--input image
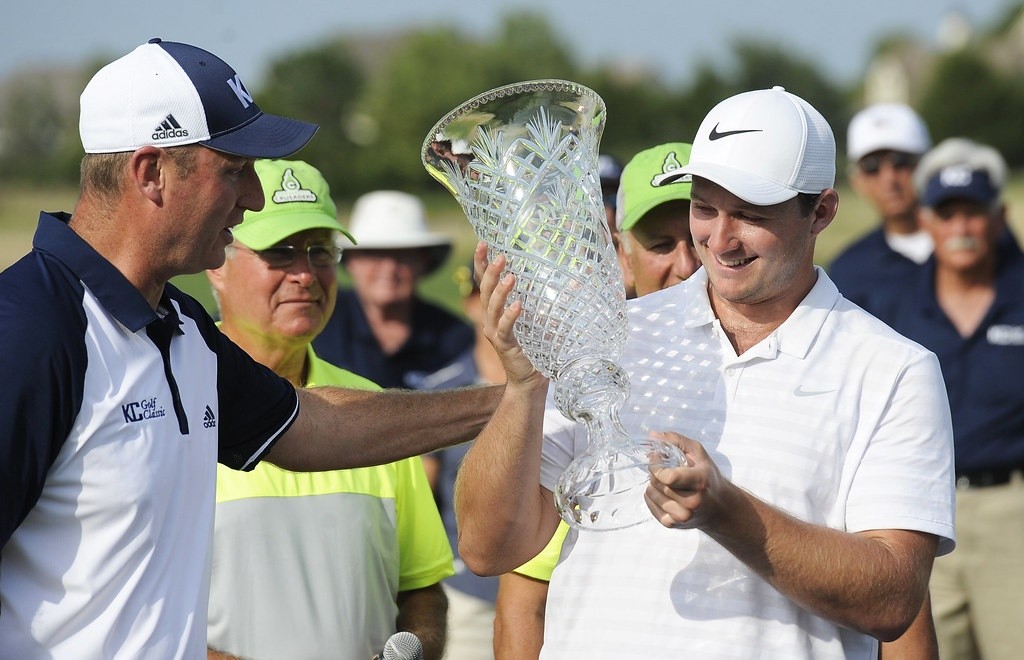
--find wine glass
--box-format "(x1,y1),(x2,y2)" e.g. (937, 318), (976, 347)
(421, 79), (688, 533)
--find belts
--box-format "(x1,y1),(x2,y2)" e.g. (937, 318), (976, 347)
(953, 468), (1021, 487)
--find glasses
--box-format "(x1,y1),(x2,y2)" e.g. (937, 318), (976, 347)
(225, 243), (344, 268)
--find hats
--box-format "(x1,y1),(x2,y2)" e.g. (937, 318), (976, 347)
(656, 86), (835, 205)
(846, 104), (929, 164)
(78, 37), (320, 160)
(615, 143), (692, 231)
(919, 166), (997, 206)
(336, 191), (456, 276)
(228, 159), (357, 252)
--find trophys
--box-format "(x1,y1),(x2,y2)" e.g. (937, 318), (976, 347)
(420, 78), (690, 534)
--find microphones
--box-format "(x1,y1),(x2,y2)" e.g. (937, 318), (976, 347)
(372, 631), (422, 660)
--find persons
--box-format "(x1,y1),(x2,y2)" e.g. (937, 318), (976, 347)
(452, 85), (956, 660)
(0, 37), (507, 660)
(207, 159), (455, 660)
(310, 141), (939, 660)
(856, 138), (1023, 660)
(824, 104), (1024, 311)
